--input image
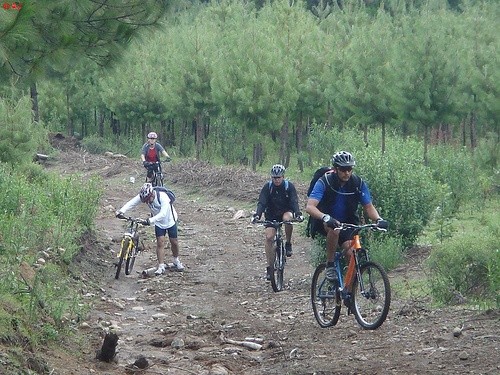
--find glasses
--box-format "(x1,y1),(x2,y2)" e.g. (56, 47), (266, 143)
(336, 166), (352, 172)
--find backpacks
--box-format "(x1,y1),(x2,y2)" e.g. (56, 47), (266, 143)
(153, 187), (176, 204)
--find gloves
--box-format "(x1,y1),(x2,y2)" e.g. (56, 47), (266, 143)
(294, 212), (304, 220)
(251, 213), (260, 223)
(115, 210), (123, 218)
(321, 214), (341, 230)
(375, 218), (387, 229)
(142, 218), (149, 226)
(143, 161), (149, 166)
(167, 156), (171, 161)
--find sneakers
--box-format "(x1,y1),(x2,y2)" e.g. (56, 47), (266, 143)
(325, 266), (338, 280)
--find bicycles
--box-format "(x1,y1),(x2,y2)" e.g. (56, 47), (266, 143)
(256, 219), (302, 292)
(114, 215), (150, 280)
(311, 223), (391, 330)
(146, 160), (170, 187)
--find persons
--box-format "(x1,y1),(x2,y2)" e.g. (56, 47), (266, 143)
(306, 151), (388, 295)
(118, 184), (185, 273)
(141, 132), (171, 181)
(250, 165), (304, 281)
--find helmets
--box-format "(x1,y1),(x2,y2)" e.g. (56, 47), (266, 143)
(271, 163), (285, 178)
(147, 132), (157, 139)
(140, 183), (152, 201)
(332, 151), (356, 167)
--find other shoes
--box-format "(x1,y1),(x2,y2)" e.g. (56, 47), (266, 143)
(285, 243), (293, 256)
(266, 268), (270, 279)
(155, 266), (165, 275)
(173, 260), (184, 270)
